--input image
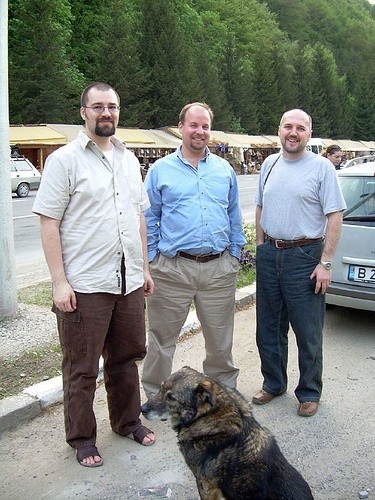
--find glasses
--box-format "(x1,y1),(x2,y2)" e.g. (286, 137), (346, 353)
(328, 145), (339, 153)
(84, 105), (121, 114)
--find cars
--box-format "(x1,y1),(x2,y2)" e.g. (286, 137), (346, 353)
(323, 155), (375, 317)
(10, 155), (41, 198)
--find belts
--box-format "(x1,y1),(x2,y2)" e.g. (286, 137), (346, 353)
(264, 233), (323, 249)
(179, 248), (228, 262)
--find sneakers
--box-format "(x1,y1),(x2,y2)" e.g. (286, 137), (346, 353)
(252, 388), (274, 405)
(298, 401), (319, 416)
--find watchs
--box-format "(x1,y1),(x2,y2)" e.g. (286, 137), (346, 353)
(319, 260), (333, 270)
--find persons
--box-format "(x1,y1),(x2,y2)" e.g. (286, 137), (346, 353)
(141, 102), (248, 401)
(32, 83), (155, 468)
(252, 109), (347, 417)
(258, 150), (264, 165)
(321, 144), (343, 165)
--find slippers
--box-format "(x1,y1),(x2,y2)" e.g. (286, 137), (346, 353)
(127, 425), (157, 446)
(75, 444), (103, 467)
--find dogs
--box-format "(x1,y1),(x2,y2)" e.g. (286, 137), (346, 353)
(137, 362), (315, 500)
